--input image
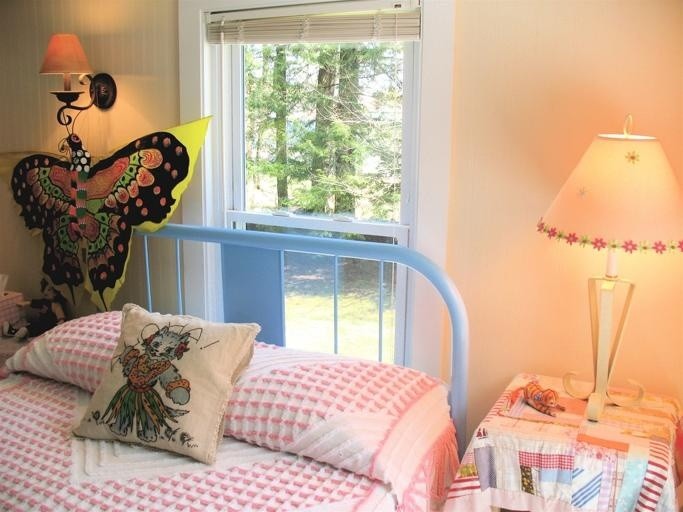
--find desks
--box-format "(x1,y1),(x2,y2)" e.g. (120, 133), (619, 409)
(473, 375), (681, 512)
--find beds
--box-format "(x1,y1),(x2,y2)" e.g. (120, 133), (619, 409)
(2, 221), (472, 512)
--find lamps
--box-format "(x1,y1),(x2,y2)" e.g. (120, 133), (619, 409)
(537, 116), (683, 409)
(39, 31), (117, 126)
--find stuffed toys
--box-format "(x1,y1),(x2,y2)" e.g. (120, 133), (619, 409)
(525, 382), (565, 416)
(3, 278), (66, 340)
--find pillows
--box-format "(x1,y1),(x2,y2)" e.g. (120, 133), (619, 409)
(67, 304), (264, 464)
